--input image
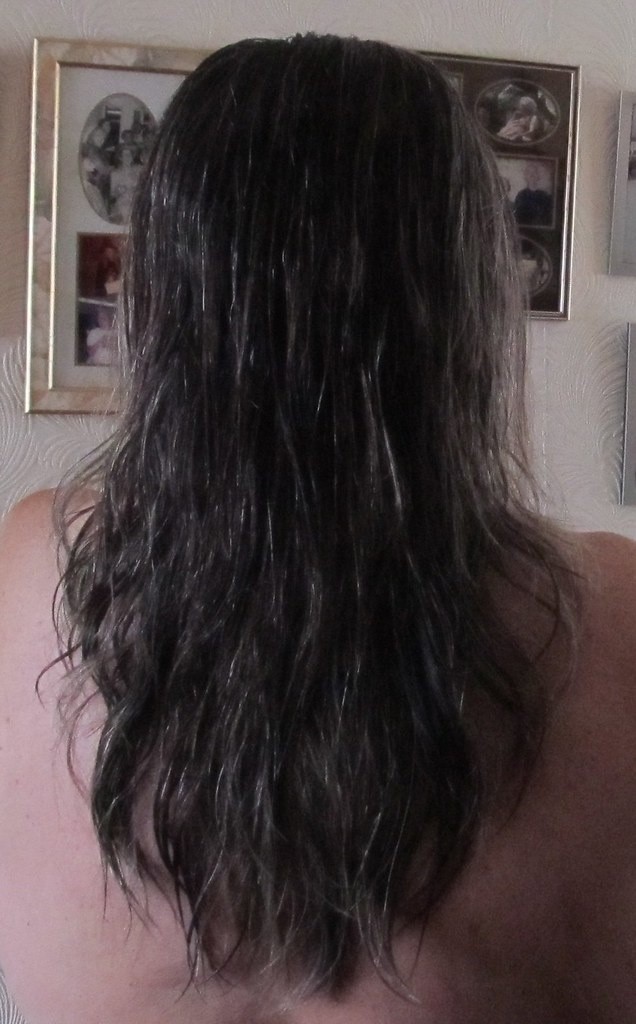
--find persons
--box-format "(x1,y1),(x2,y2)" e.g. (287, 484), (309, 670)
(83, 103), (159, 369)
(2, 31), (635, 1024)
(443, 71), (556, 307)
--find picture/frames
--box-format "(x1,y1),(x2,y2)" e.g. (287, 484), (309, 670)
(414, 45), (584, 321)
(24, 36), (215, 412)
